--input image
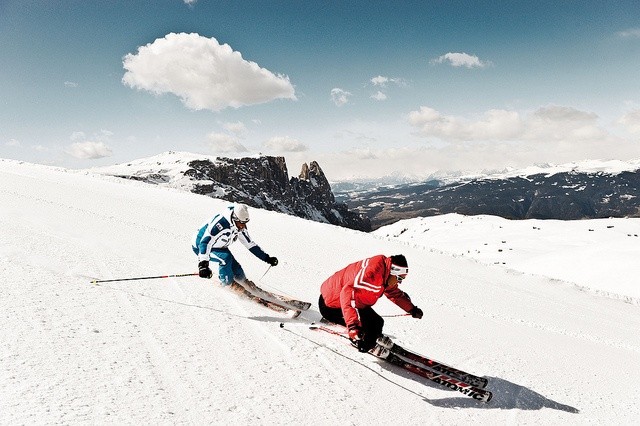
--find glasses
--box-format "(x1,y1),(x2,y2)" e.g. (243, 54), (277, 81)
(397, 275), (404, 281)
(232, 216), (246, 224)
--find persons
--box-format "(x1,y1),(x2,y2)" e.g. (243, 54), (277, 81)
(318, 254), (423, 358)
(191, 204), (278, 294)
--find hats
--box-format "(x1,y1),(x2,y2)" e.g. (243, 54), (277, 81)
(233, 204), (249, 221)
(390, 254), (408, 276)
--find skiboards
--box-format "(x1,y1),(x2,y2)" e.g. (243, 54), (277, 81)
(311, 313), (494, 405)
(210, 267), (312, 319)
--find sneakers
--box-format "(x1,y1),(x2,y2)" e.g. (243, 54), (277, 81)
(228, 281), (246, 293)
(368, 343), (390, 359)
(240, 278), (258, 290)
(376, 334), (394, 349)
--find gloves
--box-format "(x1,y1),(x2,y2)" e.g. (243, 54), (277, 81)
(266, 254), (278, 267)
(410, 306), (423, 319)
(347, 323), (365, 342)
(198, 260), (212, 279)
